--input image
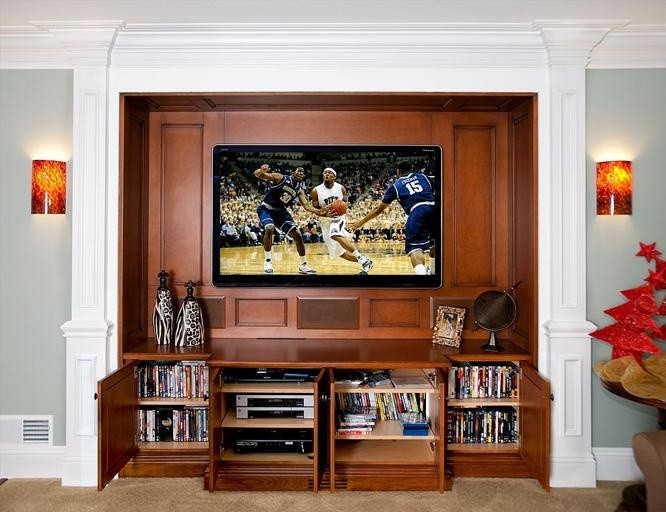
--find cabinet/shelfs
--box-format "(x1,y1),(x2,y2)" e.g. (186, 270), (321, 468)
(93, 90), (555, 495)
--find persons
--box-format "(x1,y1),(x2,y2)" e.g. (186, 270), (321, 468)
(215, 147), (438, 277)
(440, 316), (454, 338)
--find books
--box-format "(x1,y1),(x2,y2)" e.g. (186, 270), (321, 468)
(334, 361), (521, 445)
(134, 359), (209, 443)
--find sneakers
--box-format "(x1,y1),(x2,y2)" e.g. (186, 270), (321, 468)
(426, 267), (431, 275)
(358, 259), (373, 275)
(264, 258), (273, 273)
(298, 262), (317, 275)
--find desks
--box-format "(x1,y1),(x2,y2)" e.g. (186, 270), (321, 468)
(599, 377), (665, 511)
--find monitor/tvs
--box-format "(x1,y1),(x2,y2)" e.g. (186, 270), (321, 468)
(212, 144), (442, 290)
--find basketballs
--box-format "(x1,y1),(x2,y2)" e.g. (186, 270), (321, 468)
(330, 200), (347, 216)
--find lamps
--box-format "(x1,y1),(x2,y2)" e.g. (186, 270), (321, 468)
(31, 159), (68, 215)
(595, 160), (634, 216)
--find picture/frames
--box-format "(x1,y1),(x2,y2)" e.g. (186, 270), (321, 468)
(430, 304), (466, 348)
